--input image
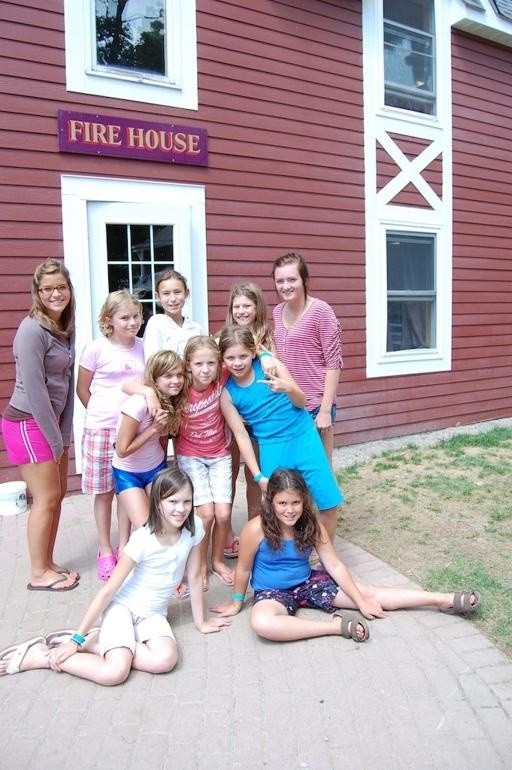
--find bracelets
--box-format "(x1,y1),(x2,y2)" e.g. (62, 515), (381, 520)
(232, 591), (245, 601)
(70, 633), (86, 648)
(259, 351), (272, 360)
(254, 473), (264, 482)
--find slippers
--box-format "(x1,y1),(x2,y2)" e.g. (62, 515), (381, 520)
(177, 536), (241, 600)
(25, 567), (80, 592)
(0, 627), (102, 676)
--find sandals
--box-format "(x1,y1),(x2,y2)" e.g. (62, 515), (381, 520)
(331, 609), (370, 644)
(440, 588), (483, 617)
(95, 545), (126, 581)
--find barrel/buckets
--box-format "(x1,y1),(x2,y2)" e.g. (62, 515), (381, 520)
(0, 480), (28, 515)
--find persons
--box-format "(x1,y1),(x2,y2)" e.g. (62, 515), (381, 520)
(271, 252), (346, 467)
(1, 257), (81, 592)
(74, 288), (146, 582)
(110, 349), (191, 601)
(219, 324), (345, 565)
(0, 467), (232, 687)
(119, 333), (280, 594)
(207, 467), (483, 643)
(221, 282), (276, 563)
(142, 269), (204, 458)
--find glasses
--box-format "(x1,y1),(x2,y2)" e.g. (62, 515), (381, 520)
(34, 284), (71, 296)
